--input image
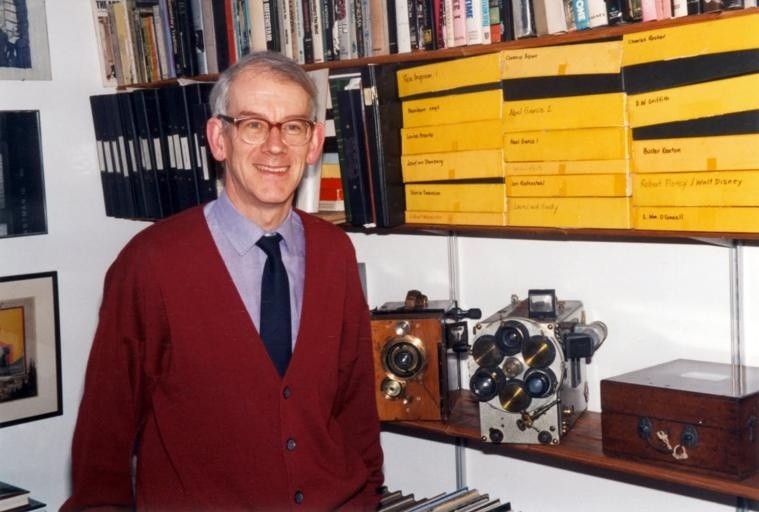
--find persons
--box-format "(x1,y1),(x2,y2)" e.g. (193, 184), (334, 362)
(52, 52), (389, 512)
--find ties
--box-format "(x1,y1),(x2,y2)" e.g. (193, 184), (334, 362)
(256, 234), (292, 376)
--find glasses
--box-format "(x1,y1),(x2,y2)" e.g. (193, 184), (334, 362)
(218, 114), (315, 148)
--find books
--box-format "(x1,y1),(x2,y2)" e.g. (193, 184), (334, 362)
(0, 479), (31, 511)
(385, 1), (759, 54)
(93, 0), (402, 233)
(379, 485), (522, 511)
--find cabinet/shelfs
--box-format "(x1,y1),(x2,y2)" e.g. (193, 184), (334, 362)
(113, 4), (759, 507)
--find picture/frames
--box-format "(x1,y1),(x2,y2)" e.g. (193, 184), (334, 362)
(0, 271), (64, 428)
(0, 0), (53, 80)
(0, 109), (48, 239)
(90, 0), (118, 87)
